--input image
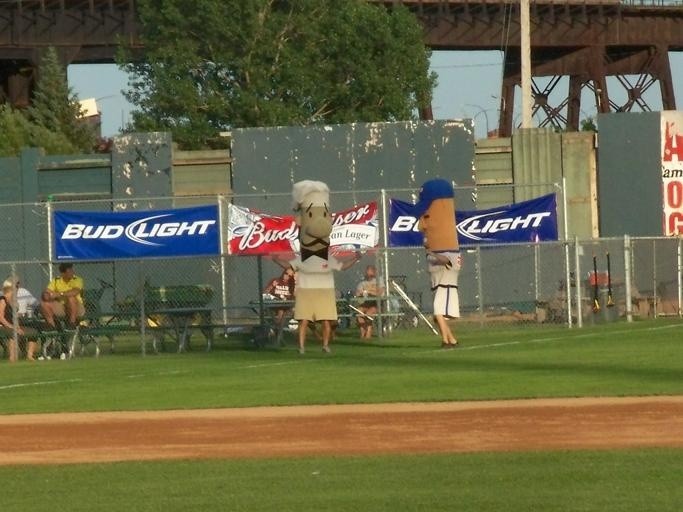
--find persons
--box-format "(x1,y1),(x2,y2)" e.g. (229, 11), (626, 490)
(39, 263), (86, 333)
(6, 275), (38, 319)
(353, 262), (384, 338)
(261, 264), (297, 346)
(411, 179), (461, 353)
(276, 178), (372, 353)
(0, 278), (38, 362)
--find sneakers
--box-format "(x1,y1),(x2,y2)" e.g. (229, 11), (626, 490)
(60, 352), (70, 359)
(441, 341), (449, 347)
(283, 271), (293, 279)
(451, 341), (460, 347)
(38, 354), (52, 361)
(297, 347), (304, 354)
(64, 326), (77, 332)
(41, 327), (58, 333)
(322, 345), (331, 353)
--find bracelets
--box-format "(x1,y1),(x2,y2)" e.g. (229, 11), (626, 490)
(59, 292), (64, 297)
